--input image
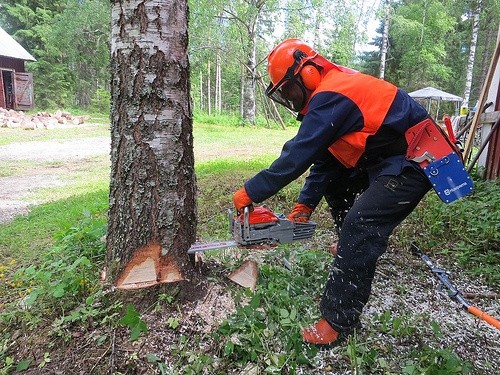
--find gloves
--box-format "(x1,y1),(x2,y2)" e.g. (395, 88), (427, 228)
(232, 188), (254, 215)
(287, 203), (313, 222)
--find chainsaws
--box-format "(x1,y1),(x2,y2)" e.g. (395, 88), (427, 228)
(187, 206), (317, 252)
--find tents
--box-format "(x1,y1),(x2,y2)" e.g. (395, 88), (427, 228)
(407, 86), (464, 123)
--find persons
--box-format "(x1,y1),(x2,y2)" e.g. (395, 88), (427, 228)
(232, 38), (476, 344)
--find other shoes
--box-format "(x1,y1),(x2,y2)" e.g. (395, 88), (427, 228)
(302, 316), (363, 350)
(330, 243), (338, 255)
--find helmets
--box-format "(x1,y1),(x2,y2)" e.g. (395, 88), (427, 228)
(268, 38), (339, 92)
